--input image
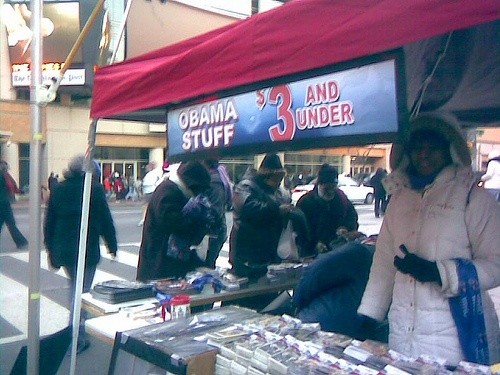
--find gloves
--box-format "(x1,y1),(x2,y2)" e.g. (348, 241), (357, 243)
(394, 243), (439, 282)
(355, 314), (378, 341)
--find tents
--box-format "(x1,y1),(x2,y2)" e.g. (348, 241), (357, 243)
(69, 0), (500, 374)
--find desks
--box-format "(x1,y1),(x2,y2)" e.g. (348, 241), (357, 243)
(82, 264), (296, 316)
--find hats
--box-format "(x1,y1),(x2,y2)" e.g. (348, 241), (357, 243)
(67, 153), (100, 178)
(259, 152), (286, 176)
(318, 163), (338, 184)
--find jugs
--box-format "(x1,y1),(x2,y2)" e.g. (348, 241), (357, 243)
(161, 294), (191, 321)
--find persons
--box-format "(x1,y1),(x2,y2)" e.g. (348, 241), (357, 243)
(381, 169), (392, 215)
(478, 150), (500, 202)
(228, 153), (305, 269)
(136, 161), (163, 227)
(136, 159), (225, 283)
(291, 233), (389, 344)
(202, 157), (233, 270)
(357, 110), (500, 371)
(161, 162), (171, 187)
(48, 172), (59, 195)
(43, 154), (119, 357)
(295, 163), (359, 253)
(0, 160), (29, 249)
(101, 172), (143, 202)
(370, 168), (387, 218)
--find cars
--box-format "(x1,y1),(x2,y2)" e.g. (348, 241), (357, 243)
(290, 176), (375, 207)
